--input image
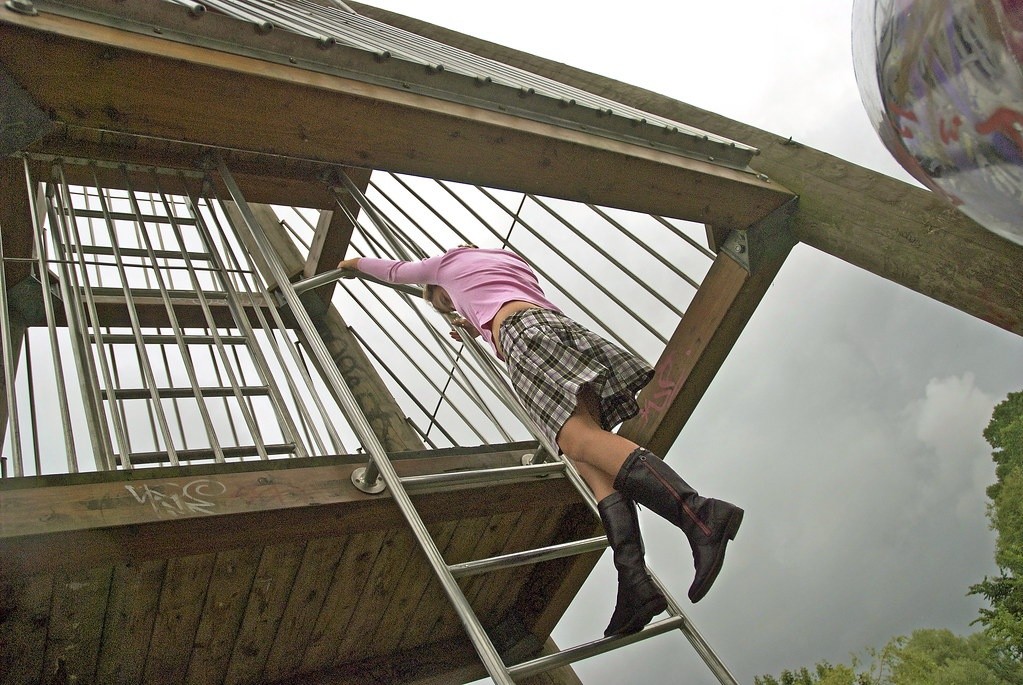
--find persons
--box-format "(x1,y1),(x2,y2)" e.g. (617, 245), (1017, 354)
(337, 244), (744, 637)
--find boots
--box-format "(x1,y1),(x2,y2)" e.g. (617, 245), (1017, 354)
(597, 492), (669, 637)
(612, 444), (744, 605)
